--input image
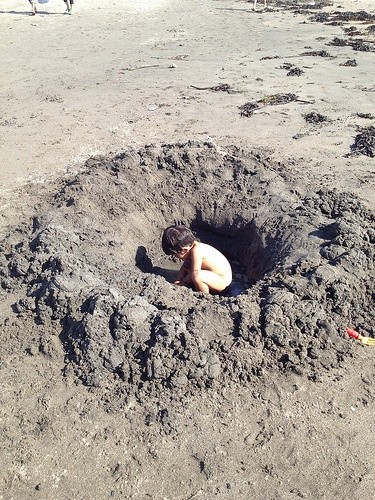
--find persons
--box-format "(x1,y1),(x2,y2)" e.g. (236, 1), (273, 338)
(161, 227), (233, 295)
(29, 0), (37, 15)
(63, 0), (74, 15)
